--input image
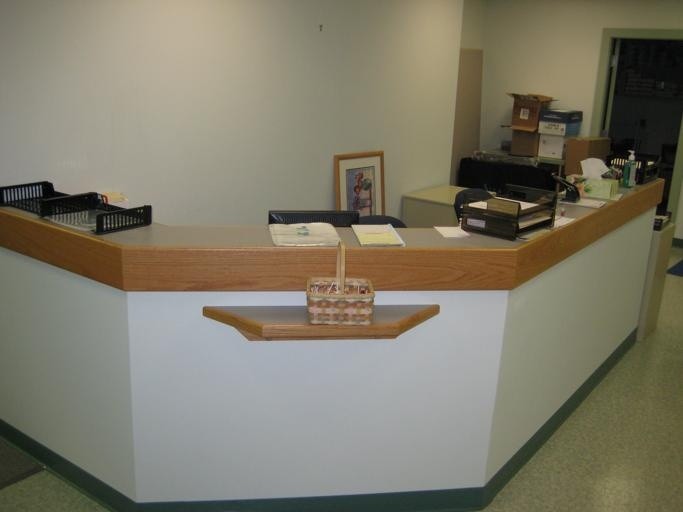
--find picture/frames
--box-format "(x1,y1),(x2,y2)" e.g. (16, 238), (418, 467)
(334, 151), (385, 219)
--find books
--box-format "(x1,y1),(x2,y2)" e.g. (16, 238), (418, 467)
(266, 220), (340, 247)
(349, 221), (406, 247)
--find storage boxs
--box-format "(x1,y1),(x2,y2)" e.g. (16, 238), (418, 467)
(565, 136), (611, 176)
(512, 128), (540, 157)
(537, 108), (583, 136)
(538, 133), (567, 159)
(507, 93), (558, 133)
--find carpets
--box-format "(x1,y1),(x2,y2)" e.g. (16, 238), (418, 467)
(0, 438), (42, 490)
(667, 258), (683, 278)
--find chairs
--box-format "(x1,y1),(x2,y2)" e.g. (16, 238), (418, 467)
(359, 215), (406, 228)
(453, 188), (493, 224)
(506, 165), (555, 201)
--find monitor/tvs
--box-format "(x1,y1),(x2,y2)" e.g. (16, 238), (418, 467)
(269, 211), (359, 227)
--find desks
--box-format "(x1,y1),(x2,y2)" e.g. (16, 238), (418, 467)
(636, 223), (675, 341)
(404, 183), (496, 227)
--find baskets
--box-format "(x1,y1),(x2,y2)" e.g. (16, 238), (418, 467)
(305, 240), (376, 327)
